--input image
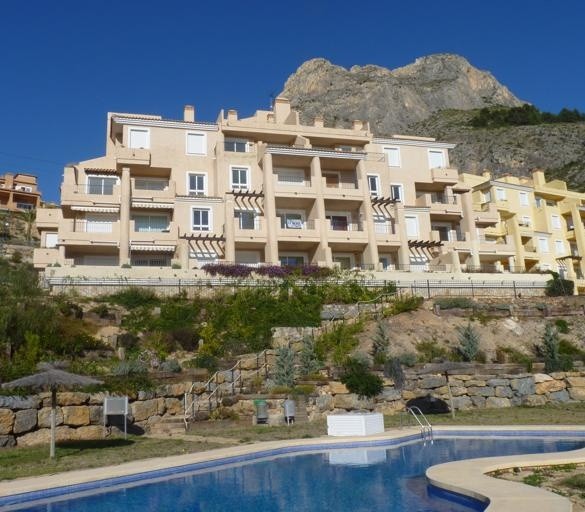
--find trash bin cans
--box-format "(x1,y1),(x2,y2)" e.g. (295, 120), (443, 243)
(283, 400), (296, 423)
(252, 399), (269, 424)
